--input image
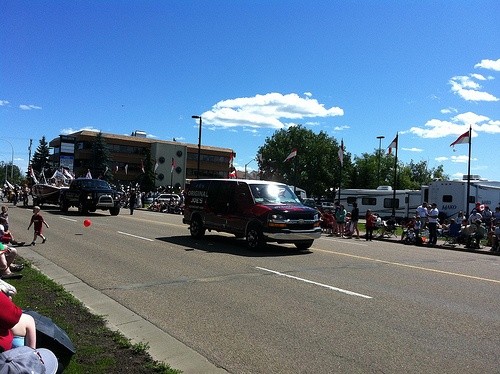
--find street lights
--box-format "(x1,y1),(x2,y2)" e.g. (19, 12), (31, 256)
(376, 136), (385, 186)
(245, 159), (256, 179)
(191, 116), (202, 179)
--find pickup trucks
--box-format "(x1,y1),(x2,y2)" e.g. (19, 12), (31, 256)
(58, 178), (121, 216)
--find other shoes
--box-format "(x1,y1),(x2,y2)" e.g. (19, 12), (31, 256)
(30, 242), (37, 246)
(10, 263), (24, 272)
(1, 272), (23, 280)
(12, 241), (25, 246)
(42, 237), (48, 244)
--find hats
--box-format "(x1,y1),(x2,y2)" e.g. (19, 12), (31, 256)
(0, 345), (59, 374)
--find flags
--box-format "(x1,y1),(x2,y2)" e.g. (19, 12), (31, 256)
(6, 180), (14, 189)
(338, 143), (343, 167)
(104, 157), (175, 175)
(283, 146), (297, 162)
(228, 152), (233, 168)
(385, 137), (397, 154)
(229, 171), (236, 177)
(30, 167), (92, 183)
(449, 131), (470, 146)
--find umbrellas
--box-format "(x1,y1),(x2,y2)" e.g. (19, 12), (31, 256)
(22, 311), (76, 354)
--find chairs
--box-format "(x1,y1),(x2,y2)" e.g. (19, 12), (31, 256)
(438, 229), (456, 244)
(372, 223), (395, 239)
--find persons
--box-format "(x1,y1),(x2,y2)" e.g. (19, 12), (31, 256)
(129, 190), (135, 215)
(365, 210), (397, 242)
(109, 182), (145, 209)
(229, 164), (238, 179)
(35, 178), (71, 187)
(27, 206), (49, 246)
(314, 202), (359, 240)
(0, 205), (25, 280)
(147, 186), (184, 215)
(0, 184), (30, 207)
(401, 200), (500, 253)
(0, 278), (58, 374)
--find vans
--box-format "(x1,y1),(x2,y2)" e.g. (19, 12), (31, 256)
(182, 178), (323, 253)
(315, 201), (336, 210)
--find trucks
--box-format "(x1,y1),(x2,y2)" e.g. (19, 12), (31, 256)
(334, 185), (420, 219)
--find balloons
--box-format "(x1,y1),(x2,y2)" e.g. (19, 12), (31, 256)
(84, 220), (91, 227)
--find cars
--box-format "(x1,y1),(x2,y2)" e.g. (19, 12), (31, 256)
(145, 194), (181, 205)
